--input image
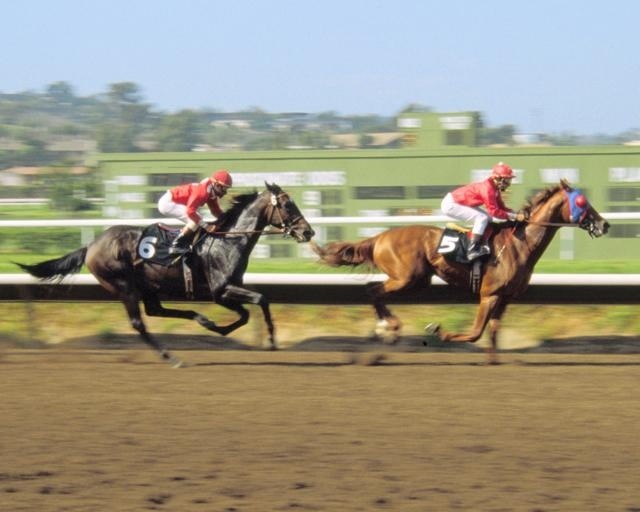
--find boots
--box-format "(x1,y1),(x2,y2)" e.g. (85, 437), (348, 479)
(168, 226), (193, 254)
(466, 234), (488, 261)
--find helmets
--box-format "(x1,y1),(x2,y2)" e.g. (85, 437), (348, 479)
(491, 162), (516, 178)
(209, 171), (232, 187)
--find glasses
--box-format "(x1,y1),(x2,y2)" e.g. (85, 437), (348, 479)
(216, 183), (227, 193)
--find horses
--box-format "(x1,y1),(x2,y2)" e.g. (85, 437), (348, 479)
(307, 176), (612, 355)
(12, 179), (315, 358)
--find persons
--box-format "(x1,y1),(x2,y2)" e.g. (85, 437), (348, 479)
(155, 168), (233, 253)
(440, 160), (525, 262)
